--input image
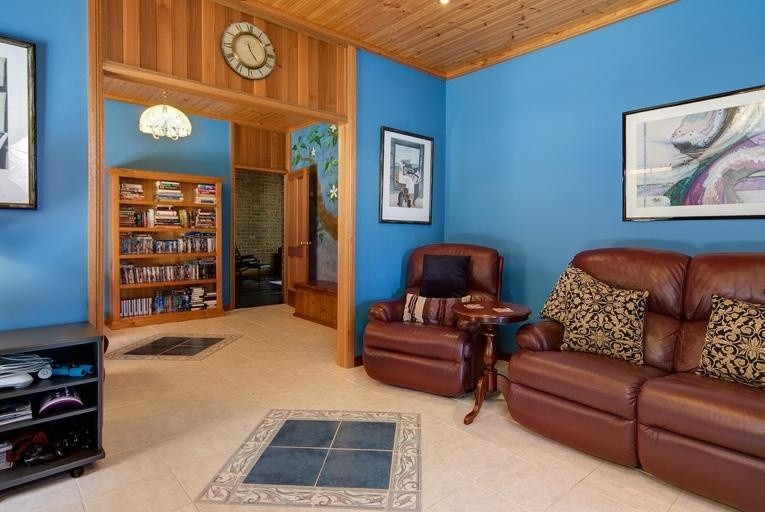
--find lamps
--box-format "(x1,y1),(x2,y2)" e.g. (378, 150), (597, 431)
(139, 91), (192, 140)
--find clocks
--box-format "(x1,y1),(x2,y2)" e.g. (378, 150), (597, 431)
(220, 21), (275, 80)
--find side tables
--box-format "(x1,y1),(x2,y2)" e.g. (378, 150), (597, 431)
(451, 301), (531, 425)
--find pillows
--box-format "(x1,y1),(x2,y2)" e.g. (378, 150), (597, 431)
(695, 294), (765, 390)
(560, 279), (650, 366)
(419, 254), (472, 298)
(401, 292), (471, 327)
(536, 264), (614, 324)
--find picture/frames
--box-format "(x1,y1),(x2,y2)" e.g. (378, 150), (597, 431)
(378, 126), (434, 225)
(0, 35), (38, 211)
(622, 84), (765, 221)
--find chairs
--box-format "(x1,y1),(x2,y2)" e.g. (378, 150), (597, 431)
(362, 244), (504, 399)
(235, 242), (261, 292)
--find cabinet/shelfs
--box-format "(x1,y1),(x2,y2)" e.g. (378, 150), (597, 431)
(104, 167), (228, 330)
(294, 281), (337, 330)
(0, 320), (104, 495)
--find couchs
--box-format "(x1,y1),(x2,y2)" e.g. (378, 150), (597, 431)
(506, 248), (765, 512)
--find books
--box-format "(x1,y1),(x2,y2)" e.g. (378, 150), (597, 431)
(119, 179), (217, 318)
(0, 441), (15, 470)
(0, 400), (33, 426)
(241, 254), (271, 269)
